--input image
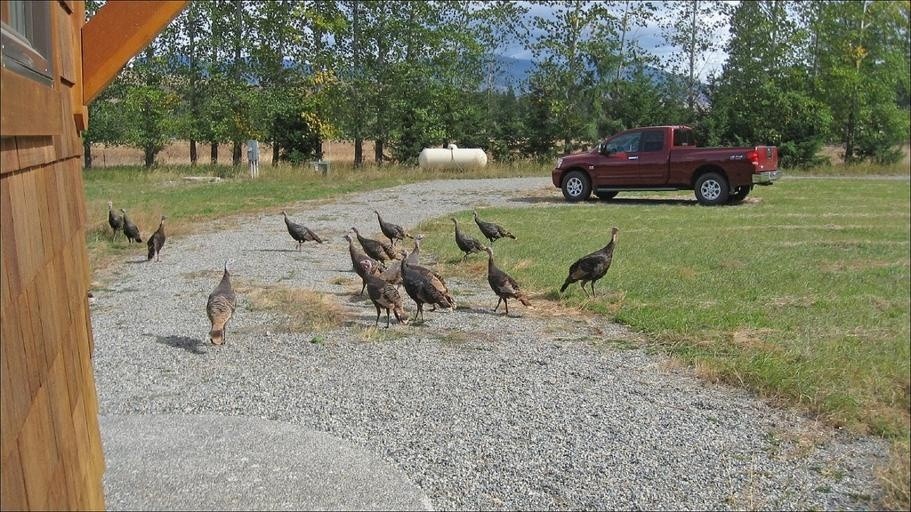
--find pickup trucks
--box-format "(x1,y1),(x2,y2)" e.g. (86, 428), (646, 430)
(551, 124), (778, 205)
